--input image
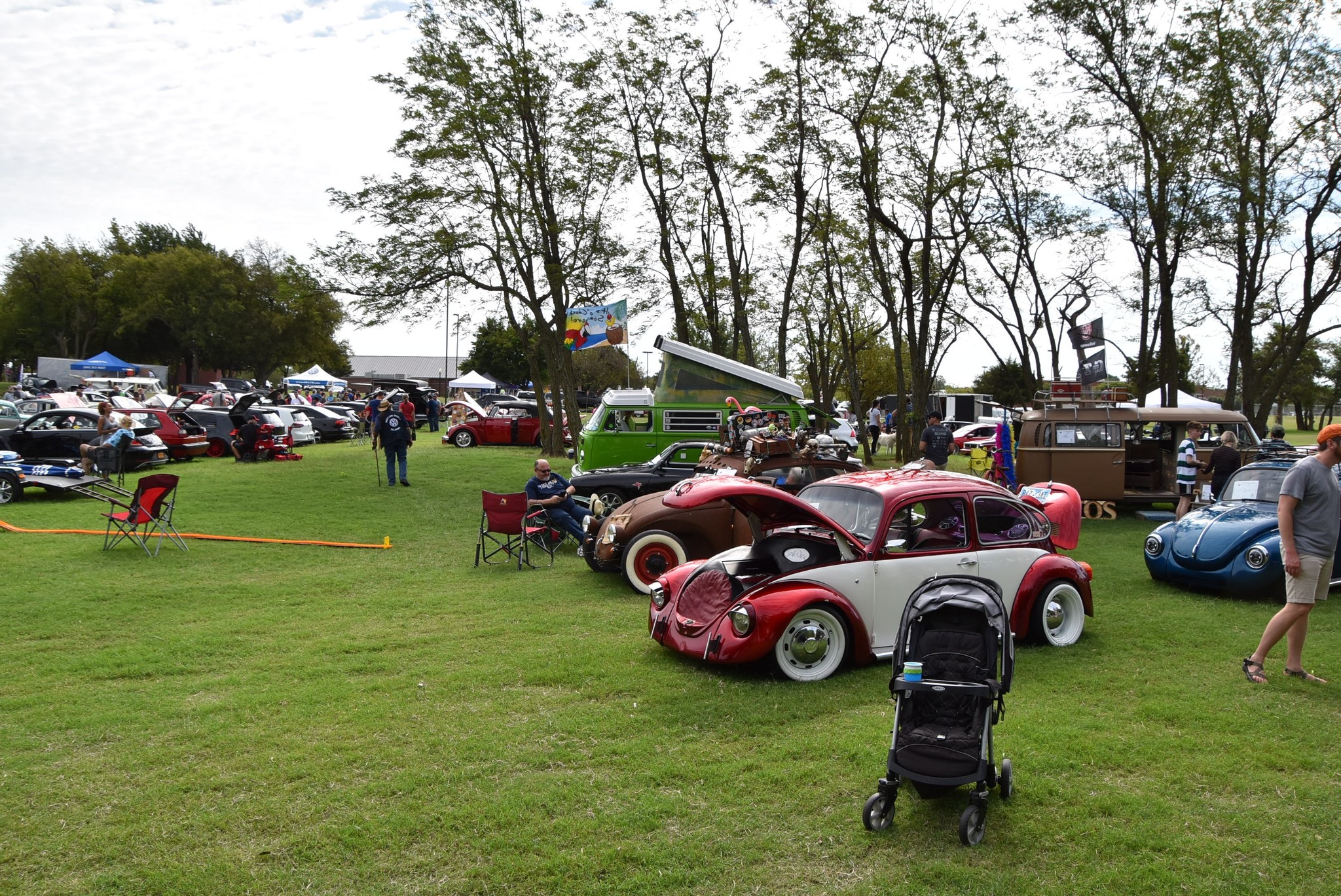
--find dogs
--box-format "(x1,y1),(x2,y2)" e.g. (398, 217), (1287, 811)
(870, 431), (902, 456)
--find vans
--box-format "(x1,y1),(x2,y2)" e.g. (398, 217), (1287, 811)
(570, 334), (814, 479)
(1015, 390), (1263, 517)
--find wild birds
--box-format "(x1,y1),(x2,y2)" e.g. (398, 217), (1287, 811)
(725, 397), (762, 414)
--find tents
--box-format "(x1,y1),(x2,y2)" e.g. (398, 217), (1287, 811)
(449, 370), (496, 398)
(1110, 383), (1222, 409)
(70, 350), (140, 381)
(283, 364), (348, 395)
(480, 372), (519, 397)
(515, 379), (534, 387)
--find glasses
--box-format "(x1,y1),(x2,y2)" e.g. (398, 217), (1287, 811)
(928, 416), (934, 420)
(305, 393), (308, 394)
(324, 395), (326, 396)
(536, 468), (552, 473)
(885, 411), (887, 413)
(345, 395), (349, 396)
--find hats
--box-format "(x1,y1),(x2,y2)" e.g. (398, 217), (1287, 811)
(15, 385), (22, 389)
(1271, 424), (1285, 434)
(906, 395), (911, 400)
(118, 387), (122, 391)
(340, 388), (353, 394)
(885, 409), (890, 412)
(377, 399), (393, 412)
(217, 386), (223, 391)
(42, 387), (47, 391)
(82, 380), (87, 382)
(106, 380), (112, 382)
(925, 411), (943, 420)
(590, 493), (605, 519)
(247, 415), (260, 421)
(118, 415), (136, 431)
(1316, 424), (1341, 445)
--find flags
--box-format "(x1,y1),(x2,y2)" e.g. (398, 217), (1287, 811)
(1066, 317), (1105, 350)
(1076, 349), (1107, 385)
(563, 299), (628, 351)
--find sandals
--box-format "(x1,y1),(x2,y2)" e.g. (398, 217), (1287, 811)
(1242, 655), (1269, 685)
(1282, 667), (1328, 685)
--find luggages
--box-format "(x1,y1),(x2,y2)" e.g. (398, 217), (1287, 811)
(719, 409), (797, 458)
(1051, 381), (1128, 403)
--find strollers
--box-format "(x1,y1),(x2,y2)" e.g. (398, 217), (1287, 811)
(862, 572), (1016, 847)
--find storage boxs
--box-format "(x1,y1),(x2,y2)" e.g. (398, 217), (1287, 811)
(748, 433), (796, 457)
(1110, 387), (1128, 401)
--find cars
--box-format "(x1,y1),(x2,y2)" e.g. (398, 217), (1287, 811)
(647, 456), (1097, 683)
(0, 368), (591, 506)
(940, 421), (976, 434)
(1143, 442), (1341, 606)
(583, 447), (872, 596)
(947, 423), (1001, 456)
(960, 433), (1002, 456)
(796, 390), (1114, 456)
(441, 400), (569, 449)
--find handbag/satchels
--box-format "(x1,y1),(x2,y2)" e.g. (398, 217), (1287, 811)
(412, 427), (416, 441)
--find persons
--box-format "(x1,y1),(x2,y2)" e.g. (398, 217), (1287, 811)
(865, 421), (871, 437)
(426, 394), (438, 432)
(247, 385), (257, 394)
(230, 415), (263, 462)
(96, 402), (121, 483)
(210, 386), (228, 407)
(1243, 423), (1341, 684)
(1253, 424), (1298, 463)
(372, 399), (413, 487)
(271, 392), (286, 406)
(784, 466), (806, 483)
(364, 396), (374, 437)
(918, 411), (955, 471)
(433, 395), (442, 431)
(1175, 421), (1209, 522)
(891, 395), (914, 453)
(399, 394), (416, 449)
(297, 387), (371, 409)
(269, 387), (274, 392)
(884, 409), (893, 434)
(592, 404), (600, 414)
(80, 415), (136, 476)
(524, 458), (594, 557)
(2, 381), (146, 408)
(865, 399), (882, 455)
(623, 411), (637, 431)
(290, 391), (305, 405)
(1201, 430), (1242, 505)
(365, 391), (386, 450)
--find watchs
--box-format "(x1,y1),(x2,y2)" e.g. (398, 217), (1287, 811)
(566, 492), (570, 498)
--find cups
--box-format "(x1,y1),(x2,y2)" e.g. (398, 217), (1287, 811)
(903, 662), (923, 681)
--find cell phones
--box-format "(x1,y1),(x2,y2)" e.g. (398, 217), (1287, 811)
(559, 491), (567, 498)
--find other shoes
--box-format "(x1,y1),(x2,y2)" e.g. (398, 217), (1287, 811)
(400, 478), (410, 486)
(372, 445), (376, 450)
(389, 481), (395, 486)
(871, 453), (878, 456)
(104, 477), (113, 483)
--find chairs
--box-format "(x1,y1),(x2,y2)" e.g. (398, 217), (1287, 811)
(474, 490), (554, 571)
(909, 538), (962, 552)
(348, 422), (371, 445)
(91, 434), (132, 491)
(242, 439), (273, 462)
(968, 446), (995, 480)
(273, 426), (293, 454)
(100, 473), (189, 559)
(526, 502), (583, 554)
(863, 526), (910, 547)
